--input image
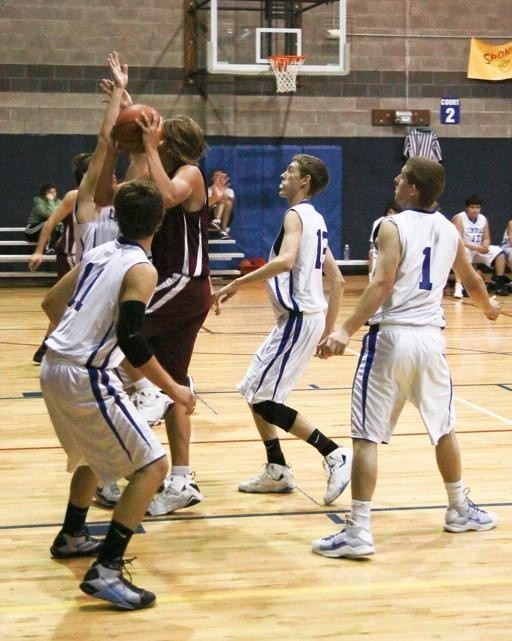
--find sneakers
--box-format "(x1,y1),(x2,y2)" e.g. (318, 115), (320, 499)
(79, 564), (157, 609)
(93, 479), (122, 508)
(49, 530), (105, 558)
(444, 506), (498, 532)
(45, 248), (55, 254)
(131, 388), (175, 428)
(494, 284), (508, 296)
(454, 284), (464, 298)
(146, 475), (202, 516)
(311, 525), (374, 558)
(207, 220), (230, 239)
(322, 447), (354, 504)
(238, 463), (296, 494)
(33, 346), (45, 361)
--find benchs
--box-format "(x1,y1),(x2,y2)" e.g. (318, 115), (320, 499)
(335, 259), (368, 266)
(0, 228), (245, 277)
(448, 272), (512, 283)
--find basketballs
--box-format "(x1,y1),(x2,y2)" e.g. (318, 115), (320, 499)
(116, 104), (161, 154)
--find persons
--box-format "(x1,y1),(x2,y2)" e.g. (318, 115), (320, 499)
(25, 50), (236, 609)
(209, 153), (354, 505)
(310, 158), (503, 560)
(450, 194), (508, 298)
(367, 203), (401, 282)
